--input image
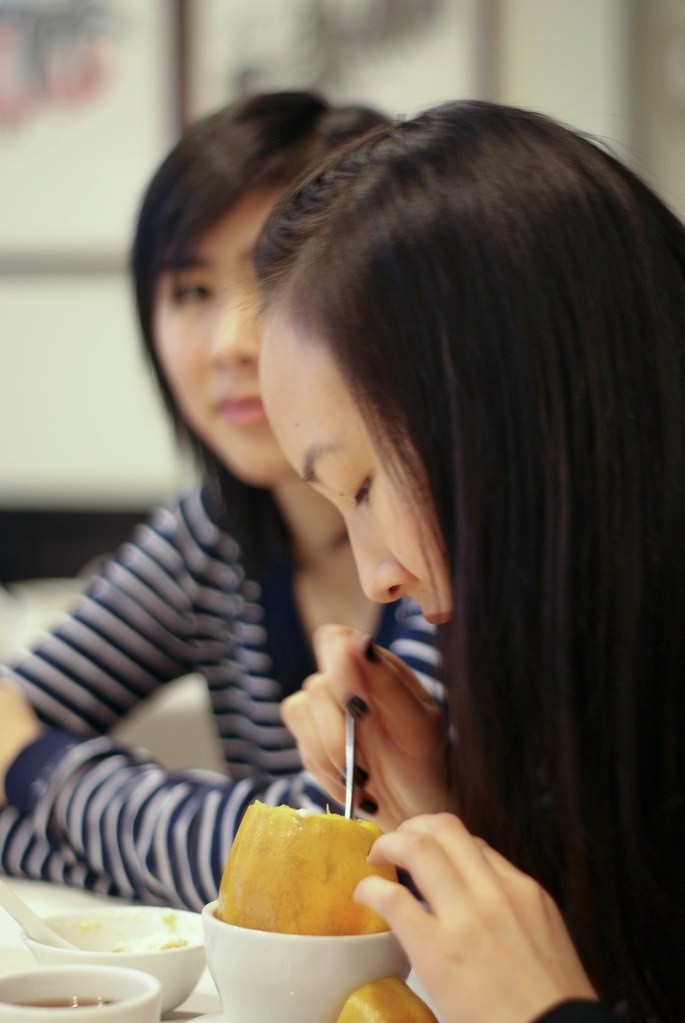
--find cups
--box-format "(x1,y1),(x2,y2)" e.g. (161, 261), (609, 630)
(0, 965), (161, 1023)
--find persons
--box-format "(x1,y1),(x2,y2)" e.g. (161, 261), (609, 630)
(0, 84), (441, 918)
(252, 95), (685, 1022)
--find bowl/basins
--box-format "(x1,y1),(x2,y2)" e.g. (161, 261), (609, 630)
(22, 907), (205, 1016)
(202, 900), (429, 1023)
